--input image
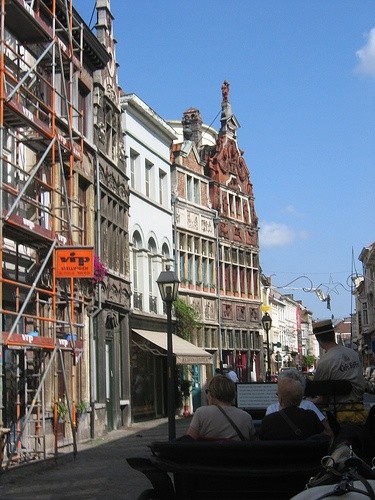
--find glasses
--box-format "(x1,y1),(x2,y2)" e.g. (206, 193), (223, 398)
(206, 389), (210, 394)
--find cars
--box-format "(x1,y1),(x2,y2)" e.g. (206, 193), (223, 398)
(362, 366), (375, 395)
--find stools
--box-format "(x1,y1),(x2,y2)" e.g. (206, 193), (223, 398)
(304, 379), (352, 419)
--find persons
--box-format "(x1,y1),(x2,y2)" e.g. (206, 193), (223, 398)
(266, 368), (332, 437)
(186, 375), (256, 441)
(308, 319), (364, 397)
(258, 377), (324, 440)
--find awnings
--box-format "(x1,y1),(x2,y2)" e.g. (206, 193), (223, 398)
(131, 328), (213, 364)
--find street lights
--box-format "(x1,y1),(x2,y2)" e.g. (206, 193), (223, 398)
(261, 311), (273, 383)
(156, 265), (182, 441)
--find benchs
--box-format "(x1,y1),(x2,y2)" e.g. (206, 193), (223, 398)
(149, 434), (333, 468)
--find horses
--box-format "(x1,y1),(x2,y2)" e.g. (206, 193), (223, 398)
(290, 404), (375, 500)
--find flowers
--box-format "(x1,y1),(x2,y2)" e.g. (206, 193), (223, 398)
(86, 254), (108, 289)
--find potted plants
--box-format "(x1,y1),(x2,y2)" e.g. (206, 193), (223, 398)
(49, 397), (87, 436)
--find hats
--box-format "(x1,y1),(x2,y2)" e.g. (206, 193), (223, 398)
(308, 319), (339, 335)
